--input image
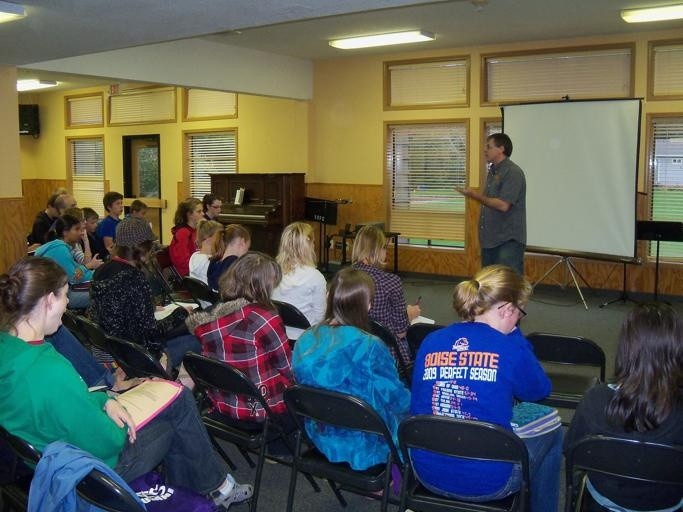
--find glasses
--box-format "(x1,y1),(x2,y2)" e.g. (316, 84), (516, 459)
(497, 301), (528, 321)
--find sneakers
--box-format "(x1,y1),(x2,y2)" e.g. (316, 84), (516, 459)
(210, 473), (253, 509)
(266, 454), (294, 465)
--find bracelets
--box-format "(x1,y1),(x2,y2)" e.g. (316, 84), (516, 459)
(482, 195), (487, 206)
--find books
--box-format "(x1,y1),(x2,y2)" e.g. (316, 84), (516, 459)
(511, 400), (562, 439)
(412, 314), (437, 328)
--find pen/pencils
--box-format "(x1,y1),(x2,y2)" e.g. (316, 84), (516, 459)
(415, 296), (422, 305)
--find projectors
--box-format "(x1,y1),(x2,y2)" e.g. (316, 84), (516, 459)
(352, 221), (386, 236)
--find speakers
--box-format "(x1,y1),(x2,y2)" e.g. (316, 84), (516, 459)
(18, 103), (40, 135)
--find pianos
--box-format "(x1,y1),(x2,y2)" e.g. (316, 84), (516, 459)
(215, 201), (281, 259)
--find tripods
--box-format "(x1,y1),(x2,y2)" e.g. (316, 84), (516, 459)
(598, 264), (640, 308)
(530, 256), (598, 311)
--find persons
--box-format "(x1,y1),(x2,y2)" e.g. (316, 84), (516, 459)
(289, 266), (411, 499)
(564, 301), (682, 511)
(25, 185), (335, 465)
(454, 132), (527, 332)
(410, 263), (564, 511)
(0, 256), (256, 507)
(348, 222), (448, 388)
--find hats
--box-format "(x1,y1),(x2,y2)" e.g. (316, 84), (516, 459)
(114, 214), (158, 248)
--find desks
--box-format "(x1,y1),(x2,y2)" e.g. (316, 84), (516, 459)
(325, 231), (401, 274)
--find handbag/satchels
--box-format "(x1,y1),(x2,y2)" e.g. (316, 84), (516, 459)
(513, 407), (562, 439)
(128, 469), (220, 512)
